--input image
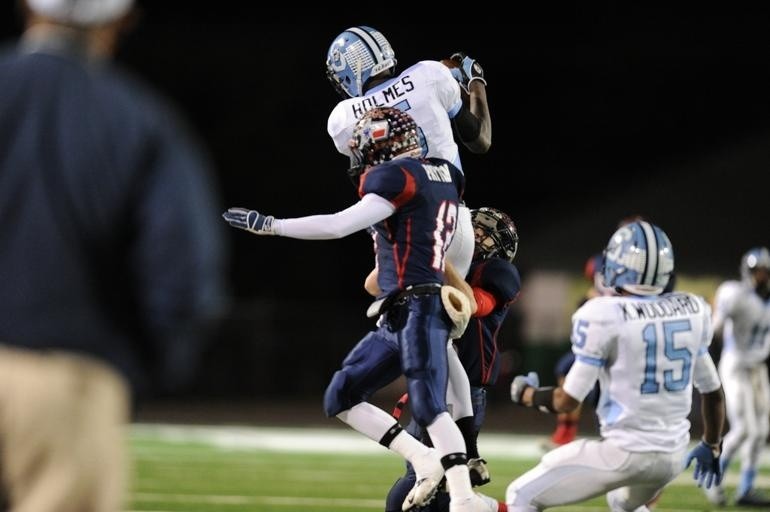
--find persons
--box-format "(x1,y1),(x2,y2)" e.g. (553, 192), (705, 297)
(324, 24), (493, 503)
(706, 246), (770, 505)
(540, 214), (663, 509)
(221, 105), (509, 511)
(0, 0), (226, 511)
(507, 221), (726, 512)
(362, 203), (522, 512)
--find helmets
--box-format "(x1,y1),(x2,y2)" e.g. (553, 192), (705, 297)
(604, 221), (675, 294)
(470, 206), (519, 263)
(348, 106), (423, 176)
(741, 248), (770, 290)
(324, 26), (399, 99)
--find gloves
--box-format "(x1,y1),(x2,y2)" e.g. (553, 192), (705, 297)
(511, 372), (539, 407)
(223, 207), (274, 236)
(684, 437), (723, 488)
(447, 52), (487, 95)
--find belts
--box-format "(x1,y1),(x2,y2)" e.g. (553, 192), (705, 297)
(389, 283), (441, 296)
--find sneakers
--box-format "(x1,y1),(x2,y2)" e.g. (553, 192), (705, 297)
(704, 476), (770, 508)
(401, 448), (507, 512)
